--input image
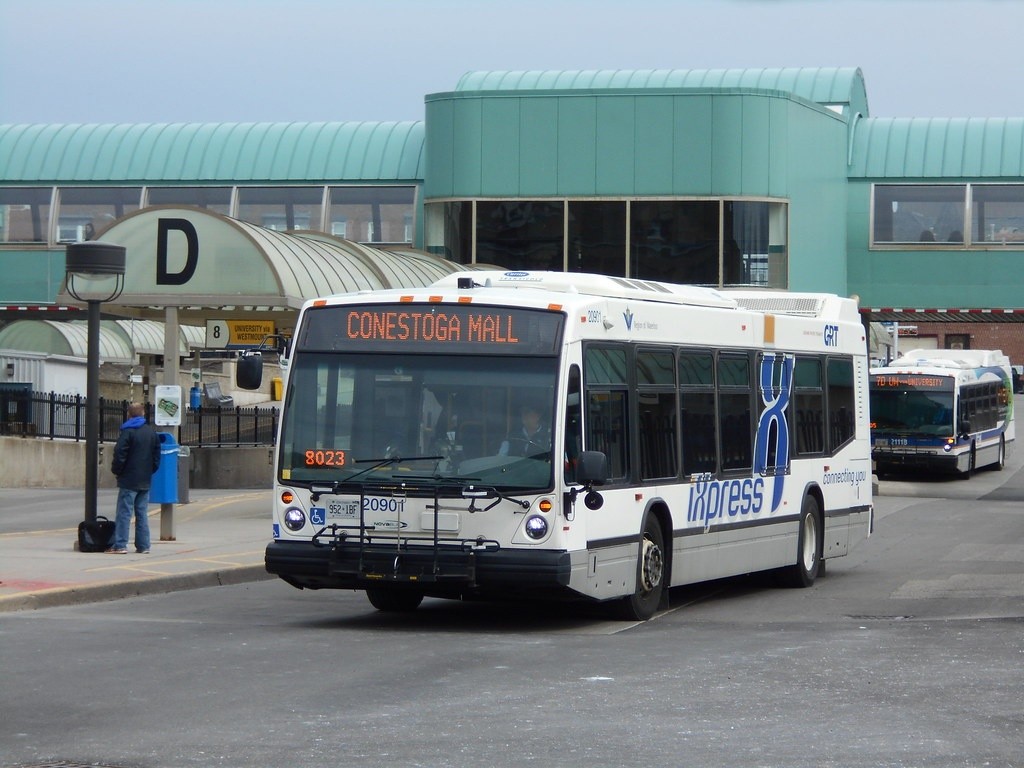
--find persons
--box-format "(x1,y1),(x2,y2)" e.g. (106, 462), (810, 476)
(499, 397), (569, 469)
(104, 402), (162, 553)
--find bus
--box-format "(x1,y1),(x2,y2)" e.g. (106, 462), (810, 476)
(870, 348), (1015, 480)
(236, 268), (871, 621)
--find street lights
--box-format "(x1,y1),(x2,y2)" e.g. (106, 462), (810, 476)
(64, 244), (127, 521)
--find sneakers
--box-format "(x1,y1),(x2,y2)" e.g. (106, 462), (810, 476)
(105, 545), (129, 554)
(135, 547), (151, 554)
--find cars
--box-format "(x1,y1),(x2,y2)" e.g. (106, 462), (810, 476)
(1011, 366), (1024, 393)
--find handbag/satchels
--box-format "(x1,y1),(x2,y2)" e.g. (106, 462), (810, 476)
(77, 515), (116, 553)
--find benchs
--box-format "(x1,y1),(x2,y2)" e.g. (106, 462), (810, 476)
(202, 381), (234, 408)
